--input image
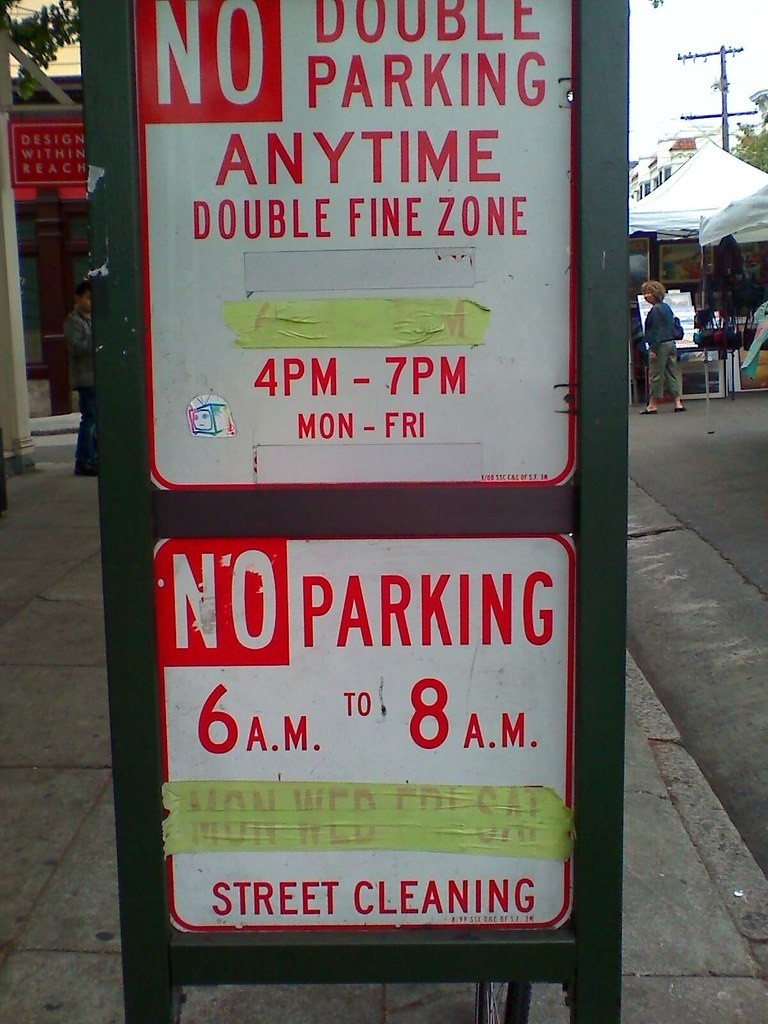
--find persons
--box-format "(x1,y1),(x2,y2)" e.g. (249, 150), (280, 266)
(640, 281), (687, 415)
(66, 280), (99, 475)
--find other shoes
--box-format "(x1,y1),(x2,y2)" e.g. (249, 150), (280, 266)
(73, 460), (99, 476)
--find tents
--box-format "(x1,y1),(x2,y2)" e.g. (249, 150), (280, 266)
(629, 140), (768, 309)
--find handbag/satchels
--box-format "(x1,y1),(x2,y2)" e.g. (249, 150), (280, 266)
(692, 308), (743, 351)
(663, 302), (684, 340)
(743, 310), (768, 351)
(67, 357), (95, 390)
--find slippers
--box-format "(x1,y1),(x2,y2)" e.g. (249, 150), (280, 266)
(674, 406), (687, 412)
(639, 408), (658, 414)
